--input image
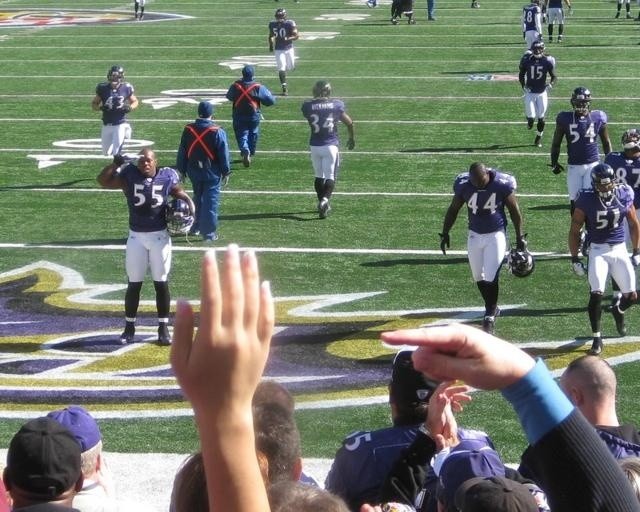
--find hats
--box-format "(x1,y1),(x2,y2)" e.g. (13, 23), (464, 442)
(8, 418), (81, 493)
(434, 443), (506, 506)
(391, 347), (439, 402)
(44, 407), (99, 454)
(454, 477), (539, 512)
(197, 102), (212, 116)
(242, 66), (253, 78)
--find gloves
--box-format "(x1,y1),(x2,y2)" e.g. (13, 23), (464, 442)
(632, 249), (640, 266)
(545, 83), (552, 89)
(551, 160), (564, 175)
(120, 105), (132, 113)
(346, 139), (356, 149)
(179, 215), (194, 233)
(517, 235), (531, 250)
(567, 7), (573, 14)
(100, 104), (113, 111)
(572, 260), (587, 276)
(522, 85), (529, 93)
(439, 234), (451, 256)
(114, 153), (144, 165)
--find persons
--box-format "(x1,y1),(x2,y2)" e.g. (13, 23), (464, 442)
(519, 41), (557, 149)
(176, 102), (231, 243)
(441, 163), (528, 336)
(615, 0), (635, 19)
(97, 149), (195, 346)
(521, 0), (543, 50)
(226, 65), (275, 167)
(92, 65), (138, 155)
(604, 128), (640, 312)
(3, 417), (85, 512)
(168, 243), (640, 512)
(391, 0), (416, 25)
(568, 163), (640, 356)
(134, 0), (146, 21)
(542, 0), (573, 43)
(47, 406), (119, 512)
(268, 8), (299, 95)
(551, 87), (611, 257)
(302, 81), (356, 219)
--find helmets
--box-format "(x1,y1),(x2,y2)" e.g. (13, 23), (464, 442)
(590, 165), (617, 186)
(509, 249), (535, 278)
(166, 200), (190, 233)
(531, 41), (544, 53)
(313, 80), (330, 98)
(106, 65), (126, 82)
(275, 9), (286, 20)
(570, 86), (592, 104)
(621, 128), (640, 146)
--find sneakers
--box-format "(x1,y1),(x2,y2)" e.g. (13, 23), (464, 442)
(615, 12), (619, 18)
(140, 13), (144, 20)
(190, 226), (199, 236)
(159, 324), (171, 344)
(391, 18), (398, 24)
(136, 14), (139, 20)
(582, 232), (591, 256)
(243, 150), (250, 167)
(486, 303), (500, 316)
(535, 136), (541, 147)
(627, 14), (633, 18)
(589, 335), (603, 354)
(578, 231), (585, 249)
(408, 20), (416, 24)
(204, 233), (218, 241)
(483, 315), (495, 335)
(613, 307), (626, 337)
(118, 327), (135, 344)
(318, 201), (328, 218)
(549, 37), (552, 42)
(282, 85), (288, 95)
(527, 118), (533, 129)
(607, 294), (622, 311)
(557, 35), (562, 43)
